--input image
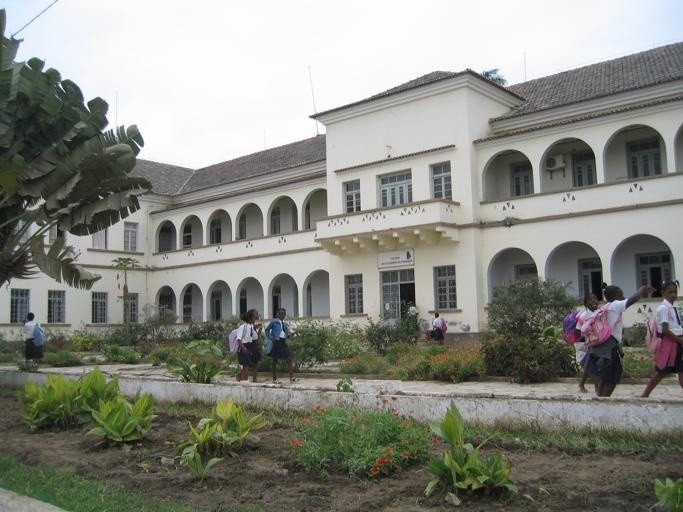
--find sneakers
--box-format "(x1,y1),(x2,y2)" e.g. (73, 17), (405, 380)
(274, 376), (282, 383)
(290, 377), (299, 382)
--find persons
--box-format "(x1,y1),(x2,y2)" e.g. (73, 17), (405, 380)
(406, 301), (419, 329)
(601, 283), (608, 303)
(432, 312), (447, 344)
(262, 307), (300, 384)
(236, 312), (261, 382)
(241, 308), (265, 380)
(640, 279), (683, 397)
(574, 291), (600, 394)
(588, 282), (657, 396)
(22, 312), (44, 371)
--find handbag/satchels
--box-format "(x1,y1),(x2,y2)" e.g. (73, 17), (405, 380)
(229, 330), (241, 353)
(262, 339), (273, 354)
(433, 327), (443, 340)
(33, 327), (44, 347)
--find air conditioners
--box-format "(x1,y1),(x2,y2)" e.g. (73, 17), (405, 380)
(544, 154), (566, 172)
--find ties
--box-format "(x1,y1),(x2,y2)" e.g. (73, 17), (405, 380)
(282, 322), (286, 332)
(674, 306), (681, 326)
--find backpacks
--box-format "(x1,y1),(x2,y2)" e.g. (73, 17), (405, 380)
(581, 302), (622, 347)
(563, 309), (587, 344)
(645, 304), (672, 352)
